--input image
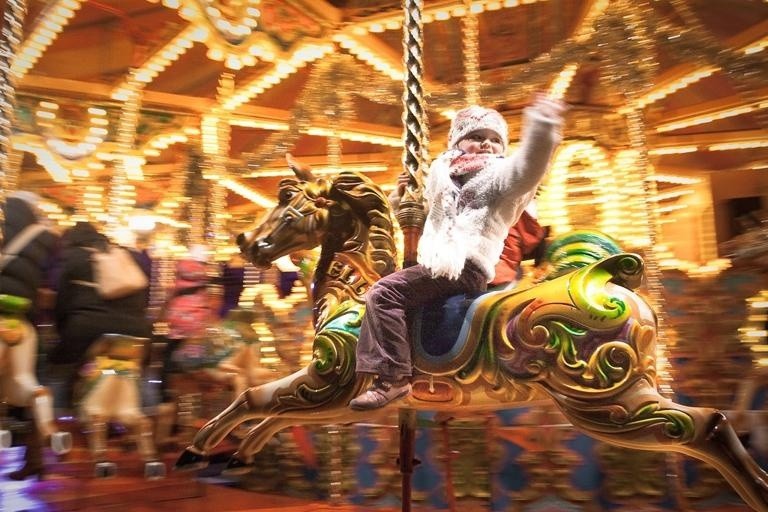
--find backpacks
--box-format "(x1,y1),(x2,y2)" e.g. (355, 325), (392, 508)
(71, 245), (149, 302)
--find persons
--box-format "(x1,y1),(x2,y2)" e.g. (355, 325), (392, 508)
(0, 197), (279, 483)
(347, 87), (564, 416)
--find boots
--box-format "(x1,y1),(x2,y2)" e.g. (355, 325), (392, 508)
(9, 421), (46, 480)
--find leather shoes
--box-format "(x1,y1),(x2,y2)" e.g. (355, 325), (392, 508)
(349, 374), (412, 411)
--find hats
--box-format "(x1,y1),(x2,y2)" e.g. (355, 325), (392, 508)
(446, 104), (510, 154)
